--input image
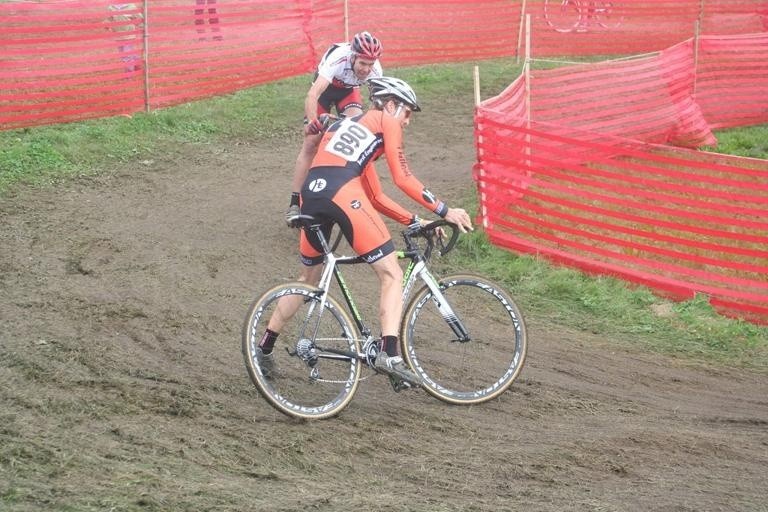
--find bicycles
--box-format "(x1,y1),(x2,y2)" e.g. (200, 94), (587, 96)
(543, 1), (625, 32)
(242, 208), (530, 422)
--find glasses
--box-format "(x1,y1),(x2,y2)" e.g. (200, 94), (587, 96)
(397, 103), (411, 118)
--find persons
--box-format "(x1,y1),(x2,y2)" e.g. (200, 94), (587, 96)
(191, 1), (224, 43)
(101, 1), (143, 83)
(286, 32), (384, 227)
(256, 76), (474, 386)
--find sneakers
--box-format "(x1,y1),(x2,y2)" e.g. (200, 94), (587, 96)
(255, 347), (285, 380)
(285, 204), (301, 229)
(375, 351), (424, 386)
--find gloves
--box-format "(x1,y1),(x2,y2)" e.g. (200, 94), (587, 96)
(305, 117), (322, 135)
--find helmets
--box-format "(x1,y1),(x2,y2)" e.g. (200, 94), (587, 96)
(349, 31), (384, 60)
(367, 77), (421, 112)
